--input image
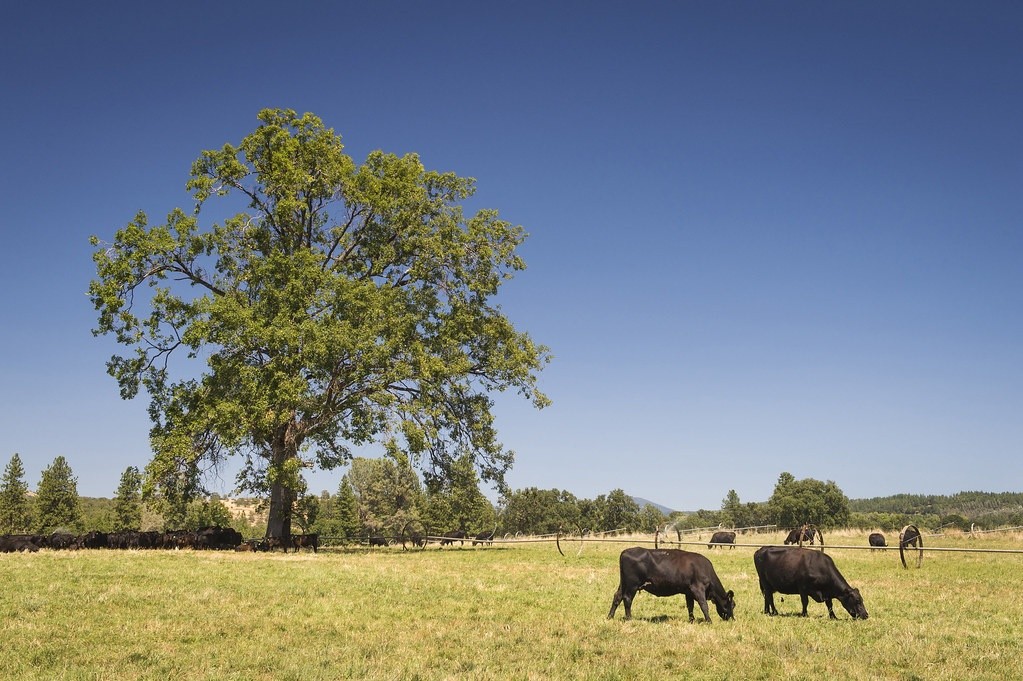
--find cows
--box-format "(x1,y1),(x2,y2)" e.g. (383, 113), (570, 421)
(608, 545), (738, 625)
(752, 545), (870, 620)
(2, 525), (496, 552)
(709, 521), (922, 548)
